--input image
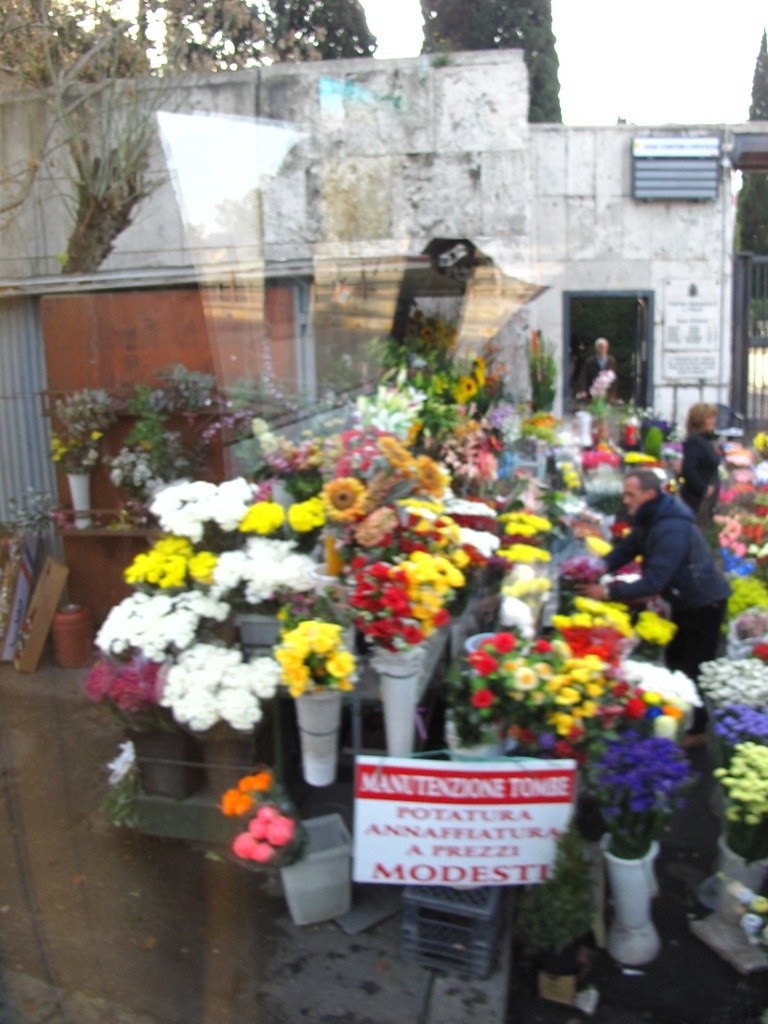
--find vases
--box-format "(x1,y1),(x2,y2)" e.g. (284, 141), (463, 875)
(717, 832), (768, 923)
(282, 812), (349, 926)
(194, 726), (245, 795)
(598, 833), (661, 965)
(237, 611), (276, 659)
(297, 691), (343, 788)
(373, 649), (424, 758)
(121, 726), (204, 801)
(65, 473), (95, 529)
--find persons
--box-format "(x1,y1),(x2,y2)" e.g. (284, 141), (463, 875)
(572, 470), (736, 742)
(578, 337), (618, 402)
(681, 403), (722, 532)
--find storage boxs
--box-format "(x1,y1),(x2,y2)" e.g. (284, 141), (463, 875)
(17, 559), (69, 673)
(400, 885), (504, 982)
(0, 525), (40, 661)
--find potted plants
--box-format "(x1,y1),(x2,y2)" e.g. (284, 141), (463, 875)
(523, 835), (596, 976)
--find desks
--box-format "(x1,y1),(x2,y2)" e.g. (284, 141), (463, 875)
(60, 530), (159, 629)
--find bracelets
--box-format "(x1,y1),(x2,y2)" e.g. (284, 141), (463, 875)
(603, 588), (609, 599)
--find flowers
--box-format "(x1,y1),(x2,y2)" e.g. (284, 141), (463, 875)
(50, 329), (768, 876)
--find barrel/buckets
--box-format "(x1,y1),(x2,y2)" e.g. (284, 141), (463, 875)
(53, 603), (93, 669)
(280, 814), (354, 927)
